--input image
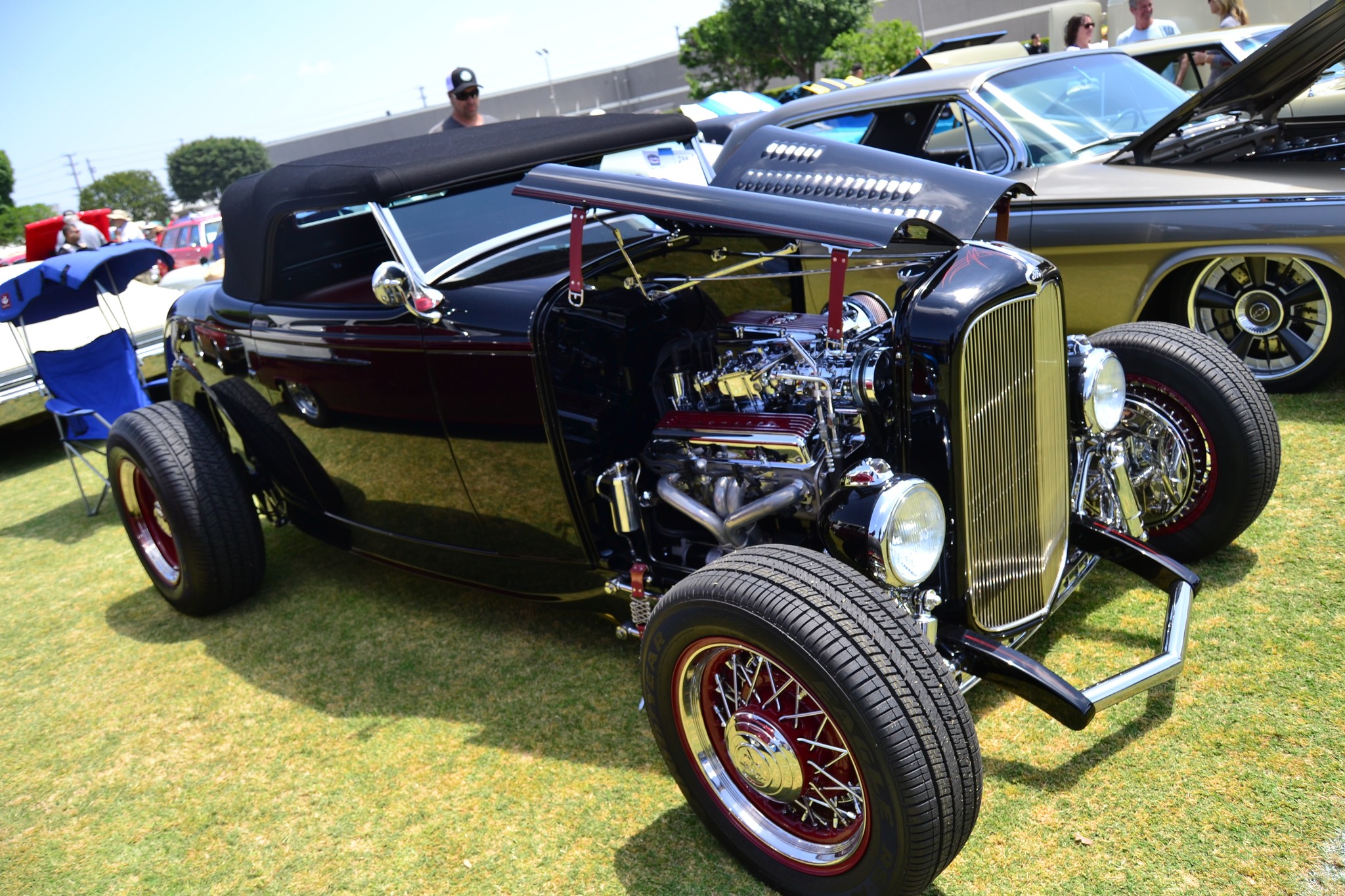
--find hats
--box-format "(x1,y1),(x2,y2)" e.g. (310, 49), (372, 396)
(105, 209), (131, 221)
(446, 67), (483, 94)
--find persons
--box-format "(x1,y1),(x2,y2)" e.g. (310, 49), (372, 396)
(55, 209), (145, 257)
(844, 63), (869, 87)
(1191, 0), (1250, 86)
(428, 67), (500, 134)
(1065, 13), (1109, 53)
(1115, 0), (1188, 88)
(1027, 33), (1048, 55)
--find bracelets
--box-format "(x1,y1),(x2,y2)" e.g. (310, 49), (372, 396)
(1204, 54), (1210, 62)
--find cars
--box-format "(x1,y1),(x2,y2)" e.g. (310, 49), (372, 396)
(106, 109), (1285, 892)
(700, 0), (1345, 394)
(927, 22), (1345, 115)
(0, 192), (226, 279)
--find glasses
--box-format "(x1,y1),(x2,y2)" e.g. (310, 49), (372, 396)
(1084, 23), (1095, 29)
(452, 89), (479, 101)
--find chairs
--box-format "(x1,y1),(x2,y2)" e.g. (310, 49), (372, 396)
(31, 327), (172, 521)
